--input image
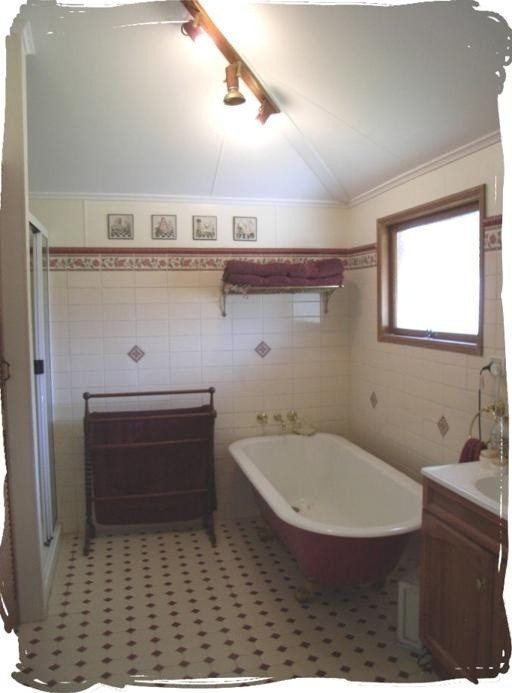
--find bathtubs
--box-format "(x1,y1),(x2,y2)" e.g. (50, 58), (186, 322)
(227, 431), (424, 587)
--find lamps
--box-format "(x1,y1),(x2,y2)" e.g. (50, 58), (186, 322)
(182, 9), (250, 106)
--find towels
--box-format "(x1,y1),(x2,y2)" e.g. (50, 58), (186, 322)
(459, 439), (487, 462)
(222, 257), (345, 289)
(84, 408), (216, 525)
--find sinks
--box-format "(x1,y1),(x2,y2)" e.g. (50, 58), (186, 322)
(417, 452), (508, 521)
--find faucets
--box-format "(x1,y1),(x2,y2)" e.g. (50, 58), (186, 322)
(273, 413), (288, 428)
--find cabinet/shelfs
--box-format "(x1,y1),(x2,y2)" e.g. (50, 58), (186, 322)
(416, 475), (510, 682)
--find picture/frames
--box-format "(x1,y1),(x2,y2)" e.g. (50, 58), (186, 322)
(232, 215), (258, 242)
(108, 213), (135, 240)
(150, 212), (178, 240)
(192, 214), (218, 241)
